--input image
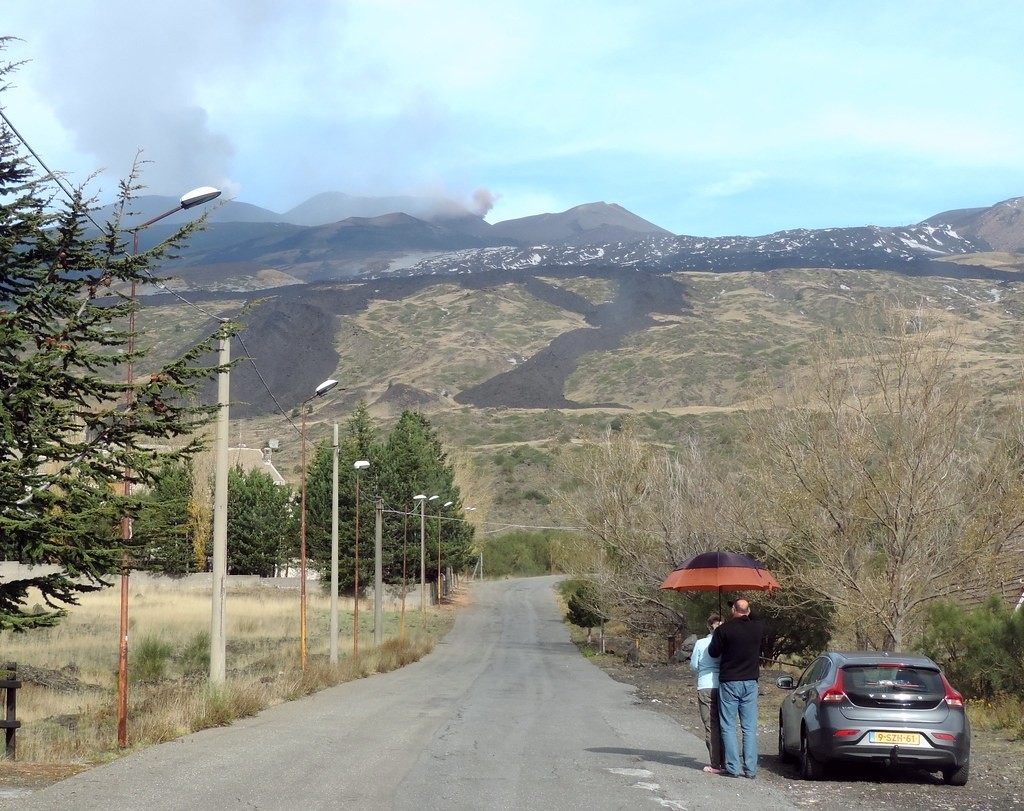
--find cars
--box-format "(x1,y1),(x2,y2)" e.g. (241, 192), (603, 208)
(777, 652), (971, 786)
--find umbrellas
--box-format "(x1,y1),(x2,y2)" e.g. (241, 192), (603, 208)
(658, 547), (782, 623)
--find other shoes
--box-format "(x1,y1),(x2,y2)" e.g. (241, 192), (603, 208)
(719, 771), (738, 778)
(703, 766), (721, 774)
(745, 774), (755, 778)
(721, 769), (726, 774)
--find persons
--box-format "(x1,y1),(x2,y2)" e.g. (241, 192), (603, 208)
(708, 598), (766, 779)
(690, 610), (726, 773)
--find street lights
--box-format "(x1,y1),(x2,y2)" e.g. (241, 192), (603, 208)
(353, 460), (370, 660)
(115, 186), (222, 749)
(410, 495), (453, 628)
(301, 380), (340, 676)
